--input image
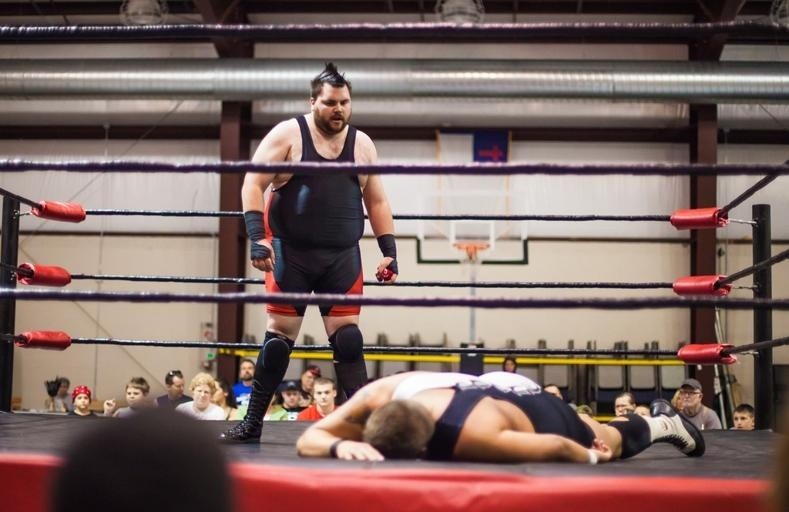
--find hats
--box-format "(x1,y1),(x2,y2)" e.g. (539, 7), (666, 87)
(680, 378), (703, 390)
(280, 380), (301, 390)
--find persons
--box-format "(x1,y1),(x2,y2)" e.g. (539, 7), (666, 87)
(217, 62), (398, 445)
(264, 365), (337, 422)
(232, 361), (255, 407)
(545, 384), (563, 400)
(177, 373), (225, 420)
(731, 403), (755, 430)
(48, 378), (74, 411)
(672, 390), (684, 411)
(68, 385), (97, 416)
(53, 409), (231, 512)
(154, 371), (193, 411)
(615, 392), (636, 416)
(635, 405), (650, 416)
(296, 371), (705, 465)
(680, 379), (722, 431)
(503, 356), (517, 373)
(213, 377), (248, 420)
(104, 377), (151, 418)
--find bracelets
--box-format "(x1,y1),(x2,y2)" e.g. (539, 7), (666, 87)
(330, 440), (346, 458)
(588, 449), (597, 464)
(244, 211), (266, 242)
(376, 234), (396, 258)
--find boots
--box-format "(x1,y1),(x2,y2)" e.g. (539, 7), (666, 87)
(640, 398), (705, 457)
(217, 377), (275, 444)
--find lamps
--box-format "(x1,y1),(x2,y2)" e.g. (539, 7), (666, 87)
(441, 0), (487, 26)
(118, 1), (170, 30)
